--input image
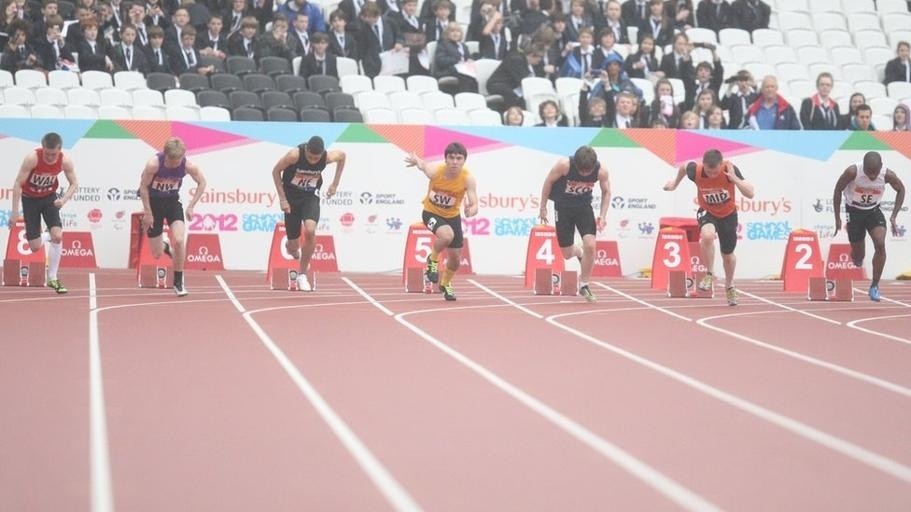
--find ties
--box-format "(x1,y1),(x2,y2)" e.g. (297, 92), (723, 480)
(340, 36), (345, 44)
(248, 43), (252, 55)
(583, 53), (588, 74)
(21, 47), (26, 59)
(638, 4), (644, 18)
(644, 56), (653, 73)
(302, 35), (309, 47)
(822, 104), (830, 124)
(503, 0), (508, 15)
(626, 120), (631, 128)
(233, 15), (238, 28)
(317, 60), (325, 75)
(256, 1), (260, 9)
(211, 40), (216, 49)
(410, 14), (417, 28)
(52, 41), (58, 62)
(598, 0), (604, 15)
(375, 25), (380, 38)
(127, 48), (131, 65)
(358, 1), (363, 9)
(187, 52), (194, 65)
(117, 8), (124, 21)
(138, 26), (147, 42)
(156, 50), (161, 66)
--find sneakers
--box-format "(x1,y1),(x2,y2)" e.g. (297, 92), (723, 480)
(173, 279), (190, 297)
(725, 285), (736, 306)
(48, 278), (69, 293)
(427, 253), (440, 283)
(852, 259), (862, 267)
(296, 274), (314, 291)
(869, 284), (880, 302)
(286, 241), (301, 259)
(163, 234), (175, 259)
(577, 245), (585, 264)
(439, 282), (458, 300)
(579, 285), (597, 302)
(699, 274), (712, 291)
(45, 228), (51, 242)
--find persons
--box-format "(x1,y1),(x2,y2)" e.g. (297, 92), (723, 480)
(404, 143), (478, 301)
(800, 72), (840, 130)
(664, 149), (755, 306)
(465, 0), (802, 130)
(539, 146), (611, 303)
(885, 41), (909, 84)
(331, 0), (471, 76)
(839, 93), (876, 130)
(8, 133), (78, 293)
(137, 137), (207, 297)
(833, 151), (906, 302)
(0, 1), (338, 80)
(272, 136), (346, 291)
(893, 103), (909, 131)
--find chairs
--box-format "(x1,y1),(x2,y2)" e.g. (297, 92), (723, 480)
(300, 108), (329, 121)
(373, 74), (406, 103)
(773, 0), (812, 27)
(785, 30), (819, 60)
(259, 56), (291, 76)
(520, 76), (559, 113)
(845, 0), (877, 25)
(163, 88), (200, 107)
(243, 74), (274, 103)
(828, 82), (852, 101)
(324, 92), (360, 121)
(596, 43), (628, 61)
(146, 72), (175, 103)
(842, 64), (877, 89)
(862, 48), (894, 70)
(630, 44), (663, 66)
(688, 47), (715, 70)
(133, 88), (163, 107)
(35, 87), (67, 105)
(853, 82), (885, 98)
(783, 98), (804, 130)
(453, 92), (495, 124)
(463, 40), (479, 52)
(0, 69), (13, 88)
(849, 13), (886, 43)
(837, 97), (853, 115)
(886, 80), (911, 103)
(112, 70), (146, 88)
(210, 73), (243, 105)
(473, 57), (502, 93)
(813, 13), (848, 45)
(426, 40), (437, 65)
(769, 61), (811, 89)
(0, 105), (29, 117)
(732, 47), (763, 73)
(419, 92), (454, 123)
(820, 30), (857, 59)
(876, 64), (887, 82)
(338, 74), (371, 94)
(503, 110), (535, 127)
(225, 56), (257, 75)
(29, 105), (62, 118)
(48, 70), (79, 87)
(628, 77), (656, 106)
(808, 61), (848, 89)
(96, 106), (131, 118)
(100, 88), (133, 107)
(0, 89), (3, 104)
(718, 27), (750, 46)
(165, 107), (198, 120)
(871, 115), (894, 130)
(432, 106), (460, 123)
(826, 47), (860, 76)
(885, 14), (910, 44)
(664, 43), (673, 54)
(463, 107), (495, 124)
(363, 107), (397, 122)
(767, 13), (776, 30)
(230, 107), (265, 121)
(626, 24), (638, 44)
(657, 78), (685, 105)
(335, 56), (357, 87)
(812, 0), (845, 26)
(199, 54), (225, 72)
(736, 61), (769, 88)
(228, 90), (266, 121)
(14, 69), (46, 87)
(779, 13), (813, 45)
(889, 31), (910, 60)
(179, 73), (210, 105)
(752, 28), (783, 48)
(865, 98), (896, 116)
(390, 92), (425, 121)
(266, 107), (296, 122)
(3, 87), (35, 105)
(308, 75), (342, 105)
(131, 107), (165, 119)
(901, 98), (911, 115)
(197, 106), (230, 121)
(855, 30), (889, 61)
(67, 88), (100, 106)
(62, 106), (97, 118)
(261, 91), (300, 120)
(714, 46), (738, 73)
(352, 92), (395, 110)
(406, 75), (444, 100)
(555, 77), (583, 127)
(877, 0), (910, 25)
(195, 90), (232, 121)
(684, 27), (716, 44)
(797, 48), (826, 76)
(398, 107), (429, 122)
(79, 69), (113, 88)
(333, 109), (361, 121)
(766, 47), (798, 76)
(786, 82), (816, 99)
(275, 74), (308, 104)
(291, 56), (303, 74)
(292, 91), (333, 121)
